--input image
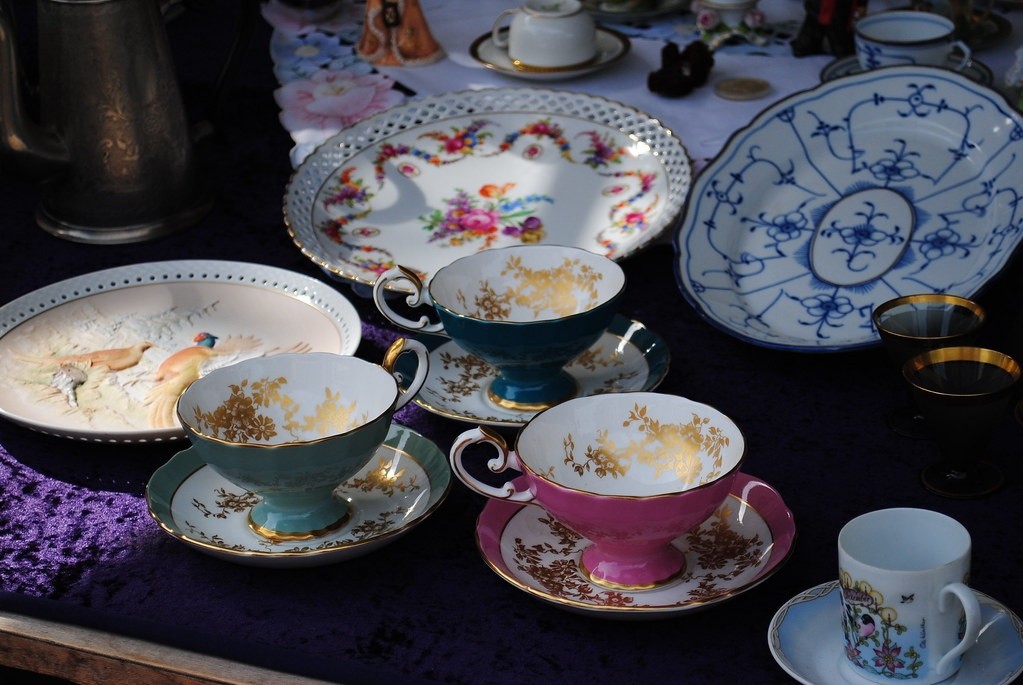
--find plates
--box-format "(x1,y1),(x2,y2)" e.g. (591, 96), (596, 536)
(884, 8), (1013, 51)
(145, 426), (450, 568)
(672, 66), (1023, 354)
(591, 0), (686, 22)
(283, 87), (692, 294)
(768, 580), (1023, 685)
(476, 471), (797, 622)
(0, 261), (361, 444)
(820, 57), (994, 87)
(394, 317), (669, 427)
(468, 26), (631, 78)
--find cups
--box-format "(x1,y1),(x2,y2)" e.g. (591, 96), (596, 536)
(492, 0), (597, 72)
(837, 508), (982, 685)
(912, 0), (992, 40)
(175, 337), (430, 541)
(449, 392), (747, 592)
(855, 11), (971, 72)
(374, 246), (627, 411)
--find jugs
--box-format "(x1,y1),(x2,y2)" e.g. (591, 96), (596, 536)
(0, 0), (257, 246)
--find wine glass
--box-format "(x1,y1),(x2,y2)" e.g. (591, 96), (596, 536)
(901, 347), (1021, 500)
(871, 294), (986, 439)
